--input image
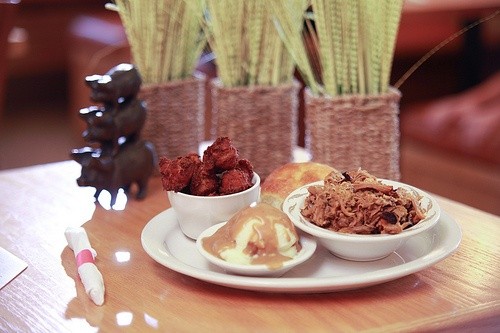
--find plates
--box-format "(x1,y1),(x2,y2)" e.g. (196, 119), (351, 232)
(195, 222), (318, 276)
(140, 207), (461, 296)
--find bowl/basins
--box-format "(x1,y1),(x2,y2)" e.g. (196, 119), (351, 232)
(282, 178), (442, 262)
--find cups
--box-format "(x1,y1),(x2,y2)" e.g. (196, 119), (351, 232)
(167, 172), (260, 240)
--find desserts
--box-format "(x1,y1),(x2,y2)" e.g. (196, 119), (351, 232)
(259, 161), (342, 209)
(202, 202), (303, 267)
